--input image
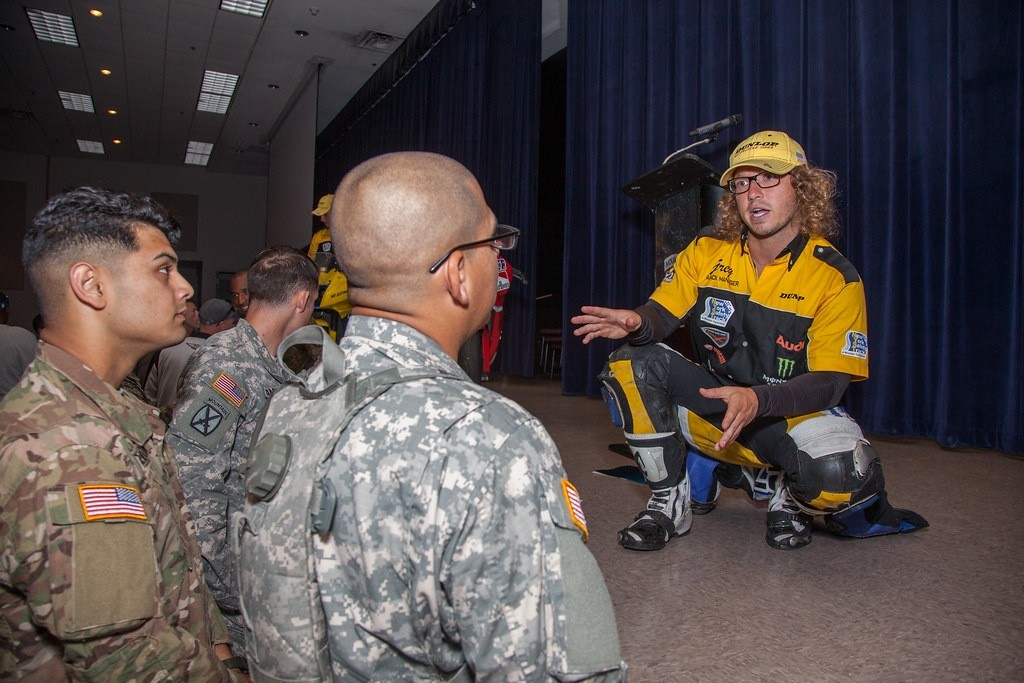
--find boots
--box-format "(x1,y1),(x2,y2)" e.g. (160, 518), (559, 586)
(618, 430), (694, 551)
(766, 470), (835, 551)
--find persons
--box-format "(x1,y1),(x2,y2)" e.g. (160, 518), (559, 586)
(570, 129), (884, 551)
(306, 194), (352, 347)
(231, 150), (630, 683)
(0, 245), (323, 658)
(0, 185), (253, 683)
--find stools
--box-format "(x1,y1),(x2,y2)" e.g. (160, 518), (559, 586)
(540, 328), (562, 379)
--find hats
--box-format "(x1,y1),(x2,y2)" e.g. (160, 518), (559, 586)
(198, 298), (235, 325)
(719, 130), (809, 187)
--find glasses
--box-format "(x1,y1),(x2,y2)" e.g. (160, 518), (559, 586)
(727, 170), (781, 194)
(429, 224), (521, 273)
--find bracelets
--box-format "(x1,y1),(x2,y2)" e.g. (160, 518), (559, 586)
(222, 656), (249, 674)
